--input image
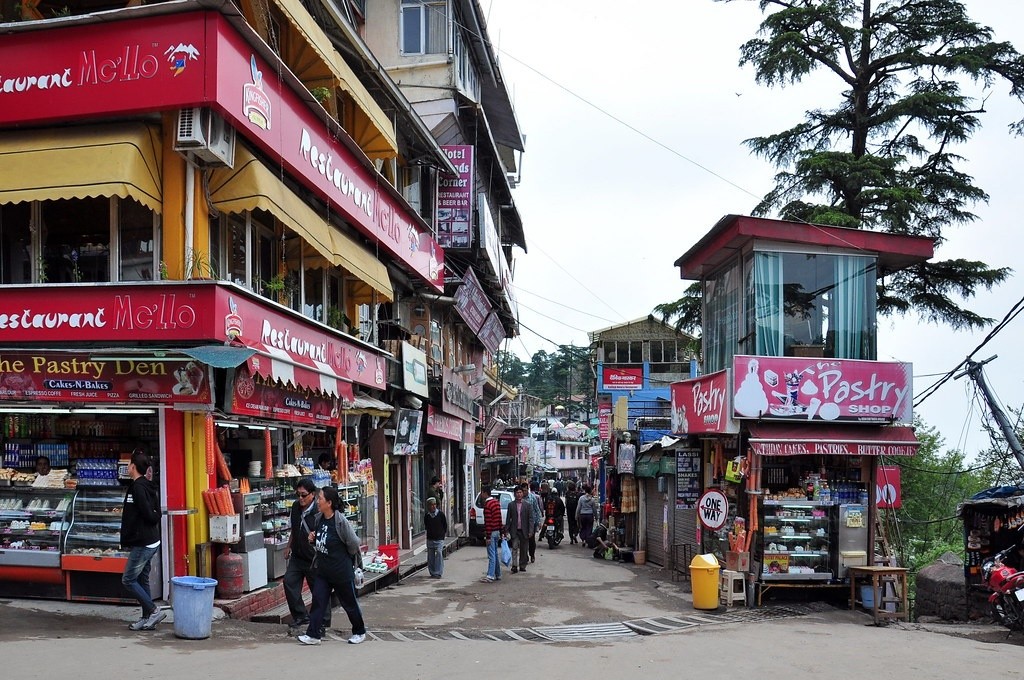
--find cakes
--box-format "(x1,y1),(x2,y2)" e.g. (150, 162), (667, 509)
(764, 526), (814, 574)
(3, 520), (70, 551)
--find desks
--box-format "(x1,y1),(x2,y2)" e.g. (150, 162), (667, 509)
(846, 564), (912, 624)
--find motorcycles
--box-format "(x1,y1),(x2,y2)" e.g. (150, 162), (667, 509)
(543, 513), (566, 549)
(980, 543), (1024, 642)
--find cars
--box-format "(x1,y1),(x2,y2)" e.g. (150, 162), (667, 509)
(469, 490), (516, 545)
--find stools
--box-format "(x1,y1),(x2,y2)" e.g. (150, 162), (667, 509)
(719, 569), (747, 609)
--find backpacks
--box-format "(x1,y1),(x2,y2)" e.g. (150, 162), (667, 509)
(548, 497), (555, 515)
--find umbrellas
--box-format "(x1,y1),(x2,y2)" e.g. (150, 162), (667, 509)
(549, 421), (590, 431)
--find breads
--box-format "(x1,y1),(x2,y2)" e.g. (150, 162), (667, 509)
(0, 467), (38, 481)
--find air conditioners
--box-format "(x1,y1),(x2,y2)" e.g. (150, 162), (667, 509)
(177, 106), (233, 167)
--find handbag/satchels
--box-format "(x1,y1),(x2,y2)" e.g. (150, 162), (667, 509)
(501, 537), (512, 567)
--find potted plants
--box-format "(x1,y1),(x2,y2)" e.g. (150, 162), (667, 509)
(173, 244), (225, 283)
(789, 333), (824, 357)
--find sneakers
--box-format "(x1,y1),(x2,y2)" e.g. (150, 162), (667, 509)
(143, 609), (166, 630)
(129, 617), (150, 631)
(348, 632), (366, 644)
(297, 634), (321, 645)
(288, 613), (310, 627)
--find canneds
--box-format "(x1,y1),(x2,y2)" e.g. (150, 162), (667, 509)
(783, 509), (805, 516)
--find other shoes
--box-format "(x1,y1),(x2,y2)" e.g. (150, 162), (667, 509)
(570, 539), (573, 545)
(519, 568), (526, 572)
(510, 566), (518, 573)
(431, 574), (441, 579)
(496, 576), (502, 580)
(480, 576), (494, 583)
(593, 552), (603, 560)
(538, 537), (542, 541)
(530, 555), (535, 563)
(582, 543), (586, 547)
(572, 534), (578, 544)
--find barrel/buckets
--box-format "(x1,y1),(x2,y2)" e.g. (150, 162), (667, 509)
(379, 545), (398, 558)
(860, 585), (881, 610)
(171, 575), (218, 639)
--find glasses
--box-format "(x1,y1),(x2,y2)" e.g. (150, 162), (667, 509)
(296, 490), (313, 498)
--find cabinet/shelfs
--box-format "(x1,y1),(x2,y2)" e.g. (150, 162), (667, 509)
(760, 499), (835, 585)
(0, 413), (366, 604)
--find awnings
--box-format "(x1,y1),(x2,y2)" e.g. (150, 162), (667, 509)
(268, 1), (399, 161)
(203, 143), (393, 306)
(0, 120), (161, 214)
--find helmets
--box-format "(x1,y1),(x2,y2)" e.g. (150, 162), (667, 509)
(551, 488), (557, 493)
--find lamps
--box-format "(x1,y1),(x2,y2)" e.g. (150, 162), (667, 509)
(453, 364), (477, 375)
(467, 374), (488, 387)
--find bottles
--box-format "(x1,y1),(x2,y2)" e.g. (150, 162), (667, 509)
(312, 469), (331, 489)
(75, 459), (120, 486)
(295, 457), (314, 471)
(799, 471), (868, 505)
(4, 412), (158, 438)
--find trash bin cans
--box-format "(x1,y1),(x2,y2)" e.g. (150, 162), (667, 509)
(170, 576), (217, 639)
(689, 552), (721, 609)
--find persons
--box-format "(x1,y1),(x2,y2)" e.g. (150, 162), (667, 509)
(617, 432), (636, 473)
(314, 453), (331, 470)
(504, 486), (534, 572)
(588, 518), (618, 559)
(298, 486), (366, 644)
(427, 476), (445, 511)
(36, 456), (50, 476)
(481, 486), (505, 583)
(424, 497), (447, 578)
(493, 474), (598, 548)
(282, 479), (320, 627)
(521, 483), (541, 563)
(120, 452), (167, 629)
